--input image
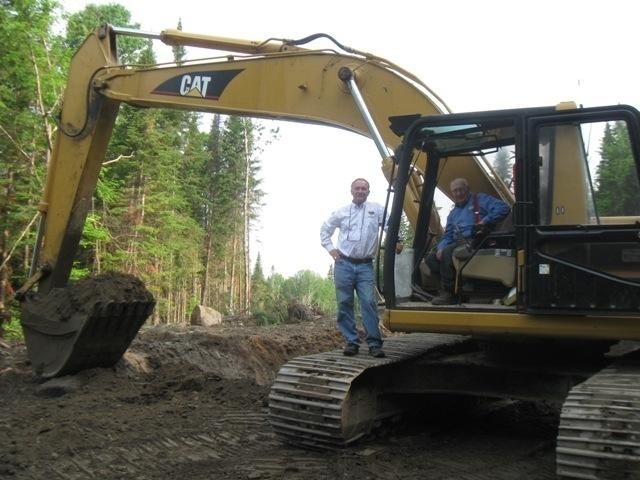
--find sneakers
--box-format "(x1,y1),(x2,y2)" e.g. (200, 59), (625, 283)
(344, 343), (360, 354)
(431, 291), (461, 305)
(369, 347), (384, 357)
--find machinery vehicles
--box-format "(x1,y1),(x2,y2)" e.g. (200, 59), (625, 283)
(14, 21), (640, 480)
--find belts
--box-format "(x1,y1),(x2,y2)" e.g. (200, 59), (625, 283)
(340, 254), (372, 263)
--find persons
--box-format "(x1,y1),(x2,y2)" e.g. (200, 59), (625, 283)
(318, 177), (406, 360)
(420, 178), (512, 308)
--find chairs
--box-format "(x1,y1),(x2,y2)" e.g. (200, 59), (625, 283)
(451, 211), (517, 288)
